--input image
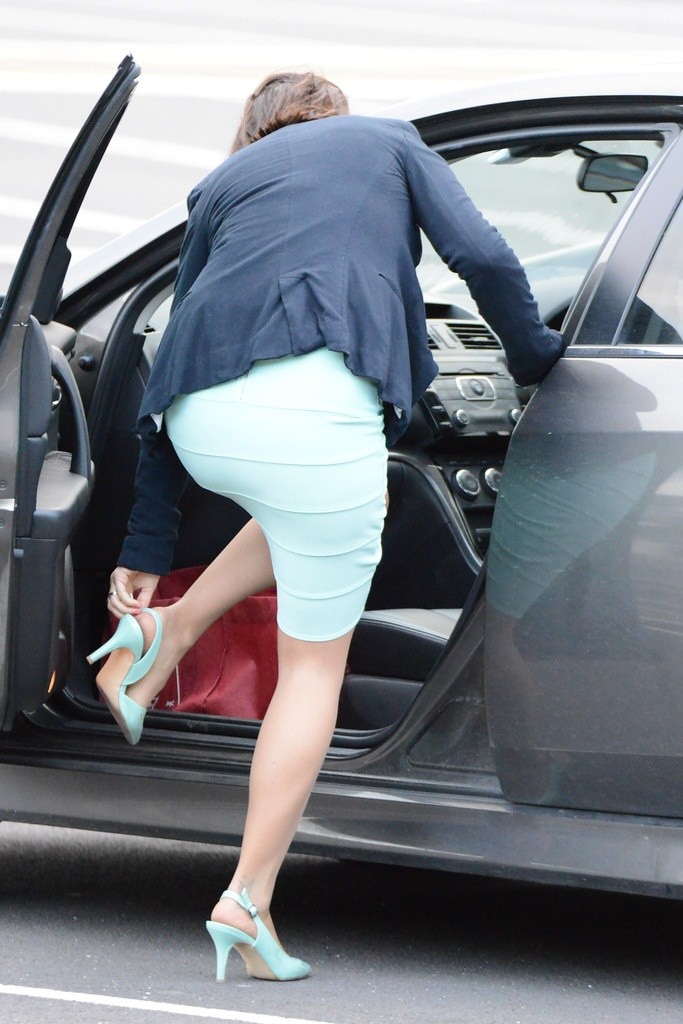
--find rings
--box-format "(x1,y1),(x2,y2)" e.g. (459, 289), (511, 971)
(109, 591), (117, 595)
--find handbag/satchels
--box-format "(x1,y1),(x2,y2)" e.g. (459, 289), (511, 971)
(106, 563), (278, 725)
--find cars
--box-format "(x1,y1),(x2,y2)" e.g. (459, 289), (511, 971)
(0, 49), (683, 915)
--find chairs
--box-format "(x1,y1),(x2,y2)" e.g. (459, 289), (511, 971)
(336, 607), (467, 727)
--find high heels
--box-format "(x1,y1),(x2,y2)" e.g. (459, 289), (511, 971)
(206, 888), (314, 985)
(85, 607), (165, 749)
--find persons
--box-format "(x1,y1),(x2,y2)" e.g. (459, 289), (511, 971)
(482, 359), (660, 750)
(84, 72), (563, 984)
